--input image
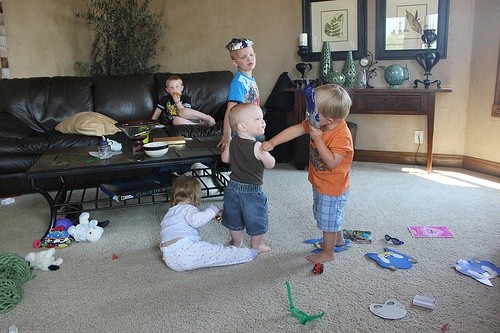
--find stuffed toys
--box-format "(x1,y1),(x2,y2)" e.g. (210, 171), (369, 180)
(26, 248), (62, 271)
(48, 212), (104, 243)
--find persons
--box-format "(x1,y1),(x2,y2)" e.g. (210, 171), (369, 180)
(218, 37), (266, 149)
(262, 84), (354, 262)
(152, 76), (217, 127)
(221, 102), (276, 251)
(159, 176), (262, 272)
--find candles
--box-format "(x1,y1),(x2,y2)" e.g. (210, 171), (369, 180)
(423, 15), (430, 28)
(298, 32), (308, 46)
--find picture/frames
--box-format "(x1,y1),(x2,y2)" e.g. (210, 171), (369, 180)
(375, 0), (450, 59)
(301, 0), (367, 61)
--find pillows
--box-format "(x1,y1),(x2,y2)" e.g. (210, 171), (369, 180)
(54, 111), (122, 136)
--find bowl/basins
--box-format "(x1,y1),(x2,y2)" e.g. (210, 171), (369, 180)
(143, 141), (168, 158)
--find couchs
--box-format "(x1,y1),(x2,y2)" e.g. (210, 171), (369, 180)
(0, 70), (234, 198)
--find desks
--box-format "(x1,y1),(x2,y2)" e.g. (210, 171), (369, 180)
(25, 137), (230, 241)
(280, 87), (453, 172)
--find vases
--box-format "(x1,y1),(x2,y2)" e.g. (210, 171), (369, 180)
(341, 50), (356, 87)
(321, 41), (332, 81)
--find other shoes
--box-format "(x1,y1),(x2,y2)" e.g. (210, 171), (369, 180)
(65, 202), (83, 223)
(72, 218), (110, 227)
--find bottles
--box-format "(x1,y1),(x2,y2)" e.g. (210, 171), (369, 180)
(98, 140), (113, 159)
